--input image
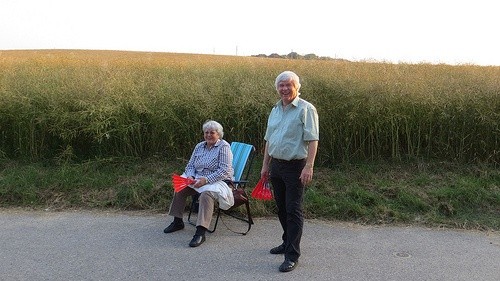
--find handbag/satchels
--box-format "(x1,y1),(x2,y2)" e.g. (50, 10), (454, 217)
(226, 180), (246, 208)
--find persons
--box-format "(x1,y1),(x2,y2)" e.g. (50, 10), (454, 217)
(164, 120), (235, 247)
(260, 71), (319, 272)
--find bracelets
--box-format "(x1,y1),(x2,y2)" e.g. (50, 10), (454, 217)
(206, 178), (208, 184)
(305, 164), (313, 168)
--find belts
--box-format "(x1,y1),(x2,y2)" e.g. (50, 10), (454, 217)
(273, 159), (292, 163)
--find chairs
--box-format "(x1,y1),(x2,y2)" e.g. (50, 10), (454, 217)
(188, 142), (256, 233)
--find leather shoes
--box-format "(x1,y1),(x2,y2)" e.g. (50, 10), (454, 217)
(270, 243), (285, 254)
(189, 233), (206, 246)
(163, 221), (184, 233)
(279, 259), (298, 272)
